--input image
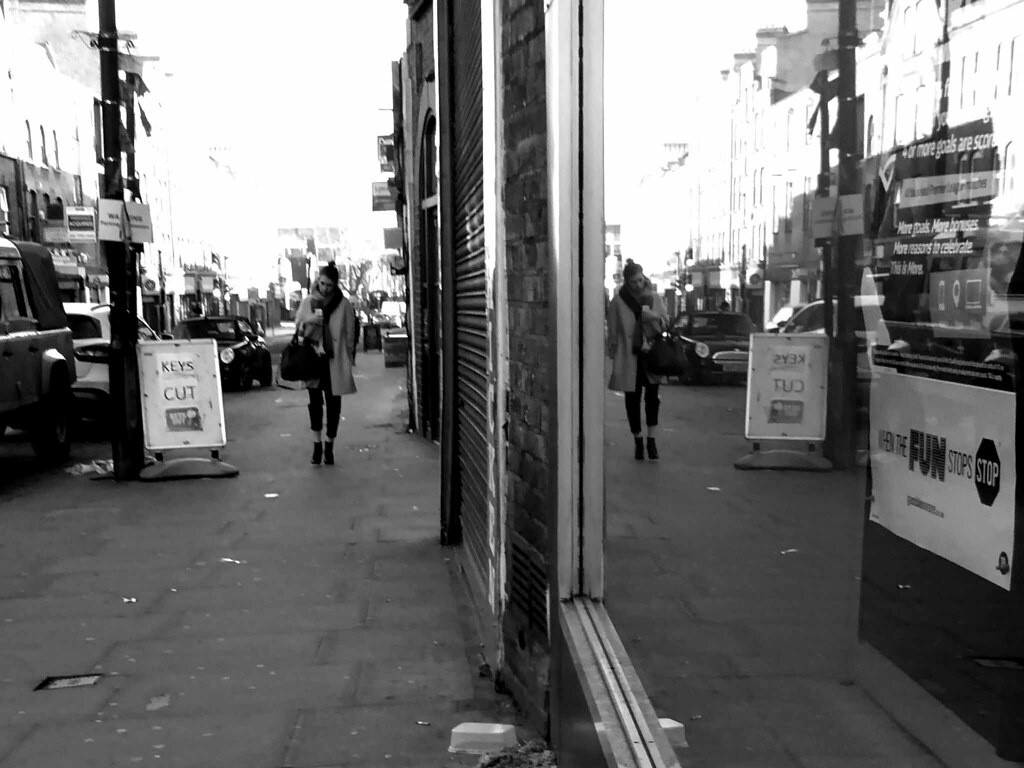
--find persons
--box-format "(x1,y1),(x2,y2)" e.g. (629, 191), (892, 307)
(607, 259), (671, 460)
(295, 261), (358, 465)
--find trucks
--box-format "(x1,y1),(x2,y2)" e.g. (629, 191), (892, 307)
(0, 237), (79, 466)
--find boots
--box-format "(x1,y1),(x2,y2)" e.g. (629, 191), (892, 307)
(647, 436), (658, 460)
(324, 441), (334, 465)
(310, 439), (323, 465)
(634, 437), (645, 460)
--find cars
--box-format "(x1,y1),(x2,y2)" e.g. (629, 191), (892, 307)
(64, 302), (174, 438)
(174, 315), (272, 391)
(780, 293), (891, 412)
(765, 303), (804, 332)
(666, 310), (760, 385)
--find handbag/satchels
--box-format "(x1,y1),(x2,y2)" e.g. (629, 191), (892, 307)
(643, 337), (689, 377)
(281, 343), (322, 382)
